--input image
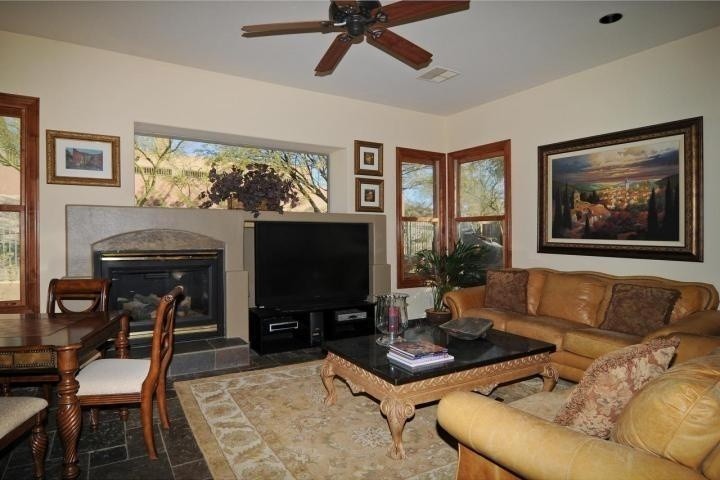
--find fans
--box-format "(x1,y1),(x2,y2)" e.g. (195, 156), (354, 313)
(241, 0), (470, 72)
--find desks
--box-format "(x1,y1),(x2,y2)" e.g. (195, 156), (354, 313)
(319, 326), (559, 460)
(0, 309), (131, 479)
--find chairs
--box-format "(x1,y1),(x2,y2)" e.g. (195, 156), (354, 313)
(0, 396), (49, 480)
(436, 331), (720, 480)
(75, 285), (184, 458)
(47, 278), (112, 313)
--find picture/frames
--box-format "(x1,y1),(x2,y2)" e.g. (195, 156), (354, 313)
(537, 115), (705, 263)
(46, 130), (121, 187)
(355, 178), (384, 213)
(354, 140), (383, 176)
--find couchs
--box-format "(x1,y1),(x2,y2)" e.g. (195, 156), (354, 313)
(444, 267), (719, 383)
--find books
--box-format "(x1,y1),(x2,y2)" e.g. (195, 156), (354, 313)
(386, 340), (454, 368)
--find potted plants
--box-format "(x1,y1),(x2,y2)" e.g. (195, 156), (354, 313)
(195, 162), (299, 219)
(412, 239), (489, 329)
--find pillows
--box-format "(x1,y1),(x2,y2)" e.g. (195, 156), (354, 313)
(484, 269), (528, 315)
(598, 283), (680, 337)
(554, 337), (680, 439)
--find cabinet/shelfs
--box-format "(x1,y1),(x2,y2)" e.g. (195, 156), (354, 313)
(249, 301), (375, 354)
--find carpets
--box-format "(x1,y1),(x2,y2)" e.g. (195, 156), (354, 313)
(173, 359), (578, 479)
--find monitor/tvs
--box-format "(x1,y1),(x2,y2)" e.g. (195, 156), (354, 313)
(254, 221), (370, 306)
